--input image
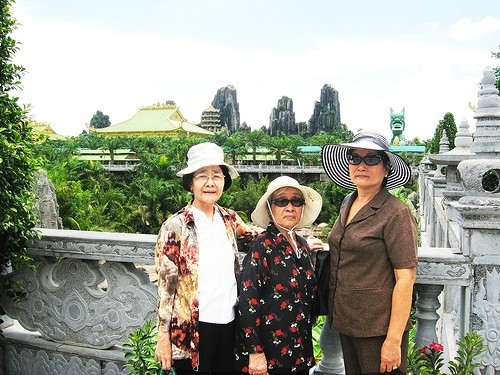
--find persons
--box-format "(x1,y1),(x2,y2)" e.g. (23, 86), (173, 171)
(322, 129), (419, 375)
(235, 177), (319, 375)
(155, 142), (323, 375)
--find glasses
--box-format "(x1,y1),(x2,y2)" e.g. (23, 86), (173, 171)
(193, 173), (226, 180)
(270, 198), (305, 207)
(346, 153), (387, 165)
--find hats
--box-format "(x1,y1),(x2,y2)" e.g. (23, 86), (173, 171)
(251, 176), (322, 229)
(322, 129), (411, 191)
(176, 143), (239, 179)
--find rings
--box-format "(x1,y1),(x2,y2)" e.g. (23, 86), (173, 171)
(392, 365), (398, 369)
